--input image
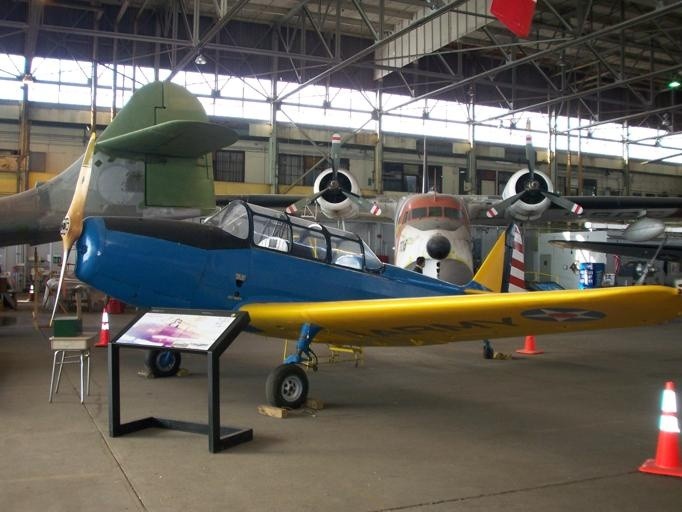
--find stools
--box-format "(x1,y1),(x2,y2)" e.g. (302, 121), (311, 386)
(47, 349), (91, 405)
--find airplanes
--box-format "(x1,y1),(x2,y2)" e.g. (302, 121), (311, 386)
(211, 130), (682, 288)
(548, 230), (682, 267)
(43, 128), (682, 413)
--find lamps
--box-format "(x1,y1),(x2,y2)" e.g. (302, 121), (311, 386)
(194, 50), (207, 65)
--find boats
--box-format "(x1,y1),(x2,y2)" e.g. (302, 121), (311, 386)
(0, 76), (242, 249)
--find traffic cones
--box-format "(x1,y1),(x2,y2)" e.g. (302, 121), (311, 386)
(637, 379), (682, 478)
(28, 283), (35, 302)
(515, 334), (545, 356)
(93, 304), (113, 348)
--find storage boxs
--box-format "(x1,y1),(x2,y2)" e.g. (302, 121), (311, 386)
(48, 332), (95, 350)
(101, 296), (125, 314)
(51, 315), (82, 337)
